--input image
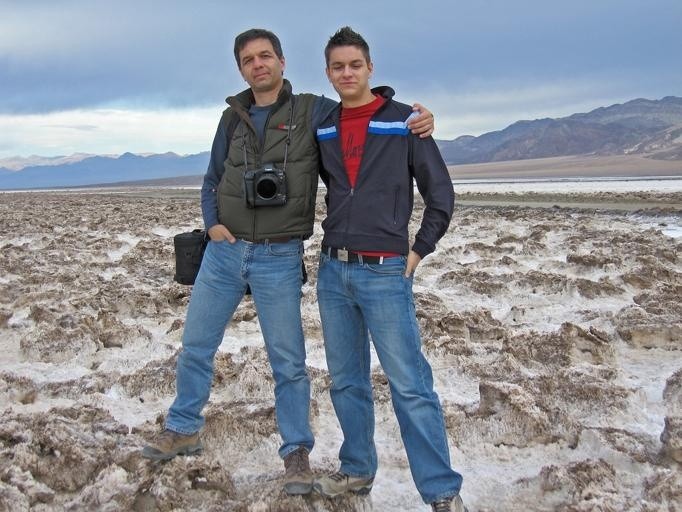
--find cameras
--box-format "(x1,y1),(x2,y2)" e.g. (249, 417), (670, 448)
(244, 168), (286, 208)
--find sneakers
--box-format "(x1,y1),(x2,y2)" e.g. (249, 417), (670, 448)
(313, 470), (374, 498)
(142, 429), (202, 459)
(284, 444), (312, 494)
(432, 493), (468, 512)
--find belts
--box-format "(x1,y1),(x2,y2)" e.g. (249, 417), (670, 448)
(238, 237), (293, 243)
(322, 245), (384, 265)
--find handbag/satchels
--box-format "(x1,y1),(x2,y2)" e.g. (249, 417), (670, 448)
(173, 229), (211, 284)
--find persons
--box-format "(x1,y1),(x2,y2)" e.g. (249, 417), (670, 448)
(311, 28), (468, 512)
(142, 28), (435, 497)
